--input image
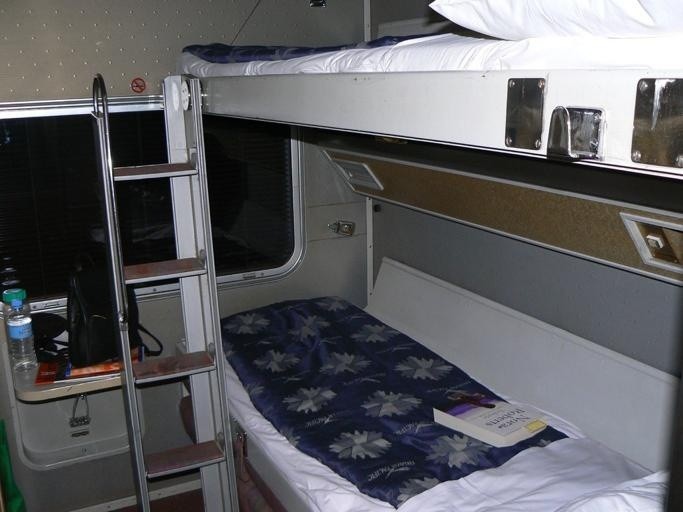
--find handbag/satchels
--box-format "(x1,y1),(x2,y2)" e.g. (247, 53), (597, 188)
(32, 314), (70, 362)
(68, 272), (163, 366)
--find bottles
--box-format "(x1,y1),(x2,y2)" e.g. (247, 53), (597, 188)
(1, 289), (38, 373)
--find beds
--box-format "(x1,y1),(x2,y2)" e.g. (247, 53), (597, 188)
(177, 290), (675, 511)
(179, 34), (683, 176)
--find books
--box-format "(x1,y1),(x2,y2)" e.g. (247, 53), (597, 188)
(64, 346), (140, 377)
(431, 387), (549, 449)
(52, 344), (146, 384)
(31, 358), (122, 386)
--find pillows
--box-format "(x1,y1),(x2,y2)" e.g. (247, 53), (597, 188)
(428, 0), (682, 36)
